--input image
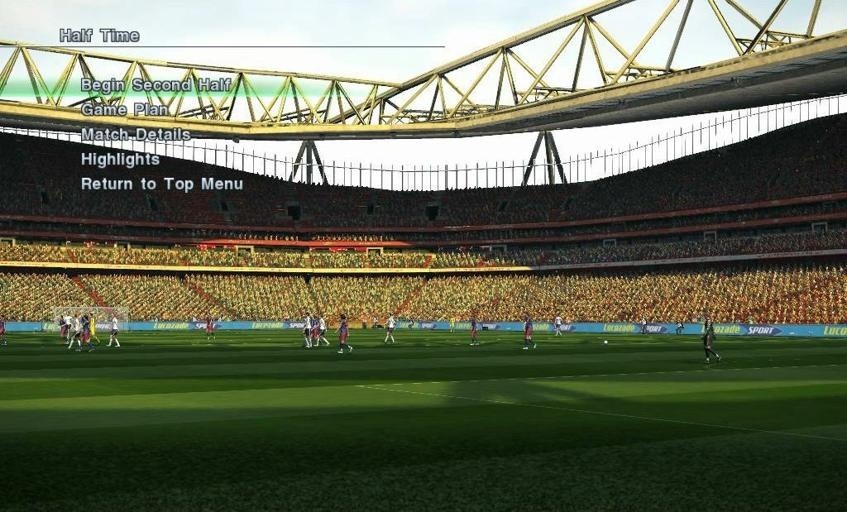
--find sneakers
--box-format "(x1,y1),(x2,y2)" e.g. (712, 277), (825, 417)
(61, 342), (120, 353)
(704, 357), (722, 364)
(304, 342), (353, 354)
(523, 344), (537, 349)
(469, 341), (480, 346)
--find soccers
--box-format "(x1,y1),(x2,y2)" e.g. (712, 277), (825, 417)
(604, 340), (608, 344)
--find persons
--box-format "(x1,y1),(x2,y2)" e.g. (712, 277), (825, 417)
(301, 309), (331, 348)
(206, 316), (216, 341)
(674, 319), (684, 336)
(554, 314), (564, 336)
(448, 316), (456, 337)
(700, 312), (720, 362)
(59, 310), (120, 352)
(642, 316), (646, 333)
(0, 110), (847, 330)
(468, 317), (480, 346)
(522, 316), (539, 349)
(382, 310), (396, 344)
(0, 321), (7, 345)
(332, 313), (355, 353)
